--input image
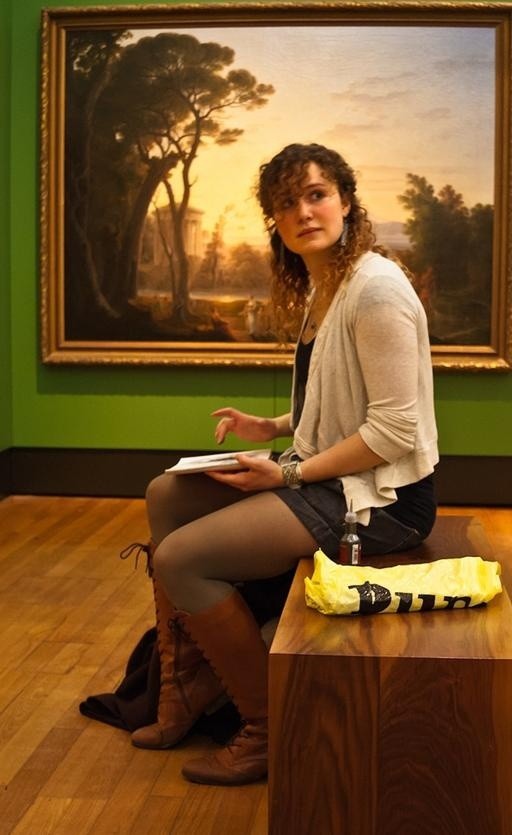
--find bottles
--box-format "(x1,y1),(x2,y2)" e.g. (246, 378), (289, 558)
(337, 511), (362, 566)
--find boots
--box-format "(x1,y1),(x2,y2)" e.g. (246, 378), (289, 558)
(170, 587), (269, 784)
(122, 538), (229, 749)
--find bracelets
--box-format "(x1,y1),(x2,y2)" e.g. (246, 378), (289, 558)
(283, 459), (304, 491)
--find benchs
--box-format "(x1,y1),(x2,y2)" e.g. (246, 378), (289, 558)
(263, 513), (511, 835)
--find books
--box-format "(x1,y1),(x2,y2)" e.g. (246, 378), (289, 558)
(163, 449), (275, 477)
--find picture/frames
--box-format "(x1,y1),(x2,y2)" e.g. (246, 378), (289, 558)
(31, 0), (511, 374)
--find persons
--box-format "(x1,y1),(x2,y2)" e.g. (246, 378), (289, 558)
(130, 142), (440, 786)
